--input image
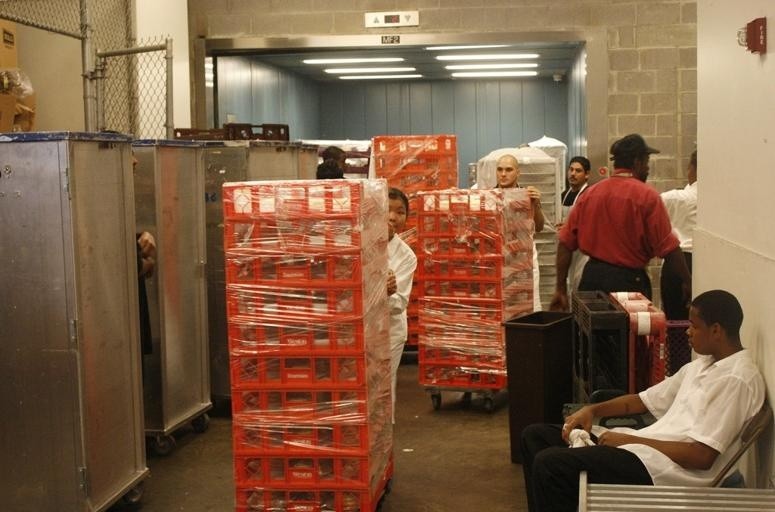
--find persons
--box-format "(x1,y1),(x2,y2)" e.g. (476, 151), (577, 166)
(491, 154), (546, 312)
(386, 184), (418, 428)
(560, 156), (592, 298)
(103, 129), (156, 359)
(523, 291), (766, 511)
(549, 132), (692, 311)
(656, 141), (695, 376)
(319, 144), (348, 174)
(315, 160), (344, 179)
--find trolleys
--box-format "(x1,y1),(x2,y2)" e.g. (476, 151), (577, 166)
(425, 389), (502, 414)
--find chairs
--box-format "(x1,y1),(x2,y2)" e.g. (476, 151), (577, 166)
(562, 389), (773, 485)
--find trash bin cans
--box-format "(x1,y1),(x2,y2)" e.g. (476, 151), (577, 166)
(499, 310), (572, 463)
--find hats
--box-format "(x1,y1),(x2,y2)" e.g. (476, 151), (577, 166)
(609, 133), (660, 161)
